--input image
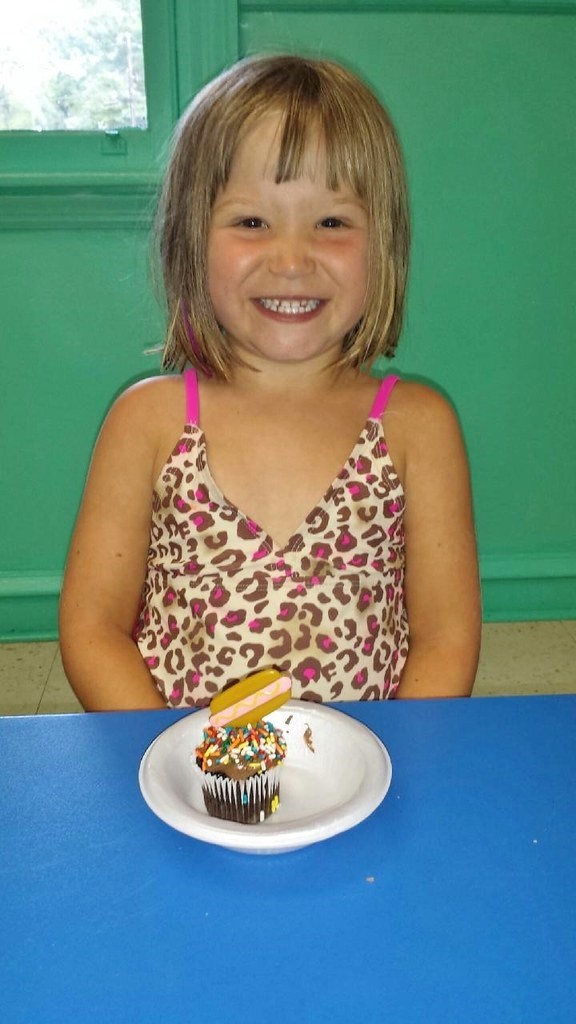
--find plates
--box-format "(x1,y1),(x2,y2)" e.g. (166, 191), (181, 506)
(137, 698), (394, 856)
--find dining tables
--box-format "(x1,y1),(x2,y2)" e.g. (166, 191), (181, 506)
(0, 693), (576, 1023)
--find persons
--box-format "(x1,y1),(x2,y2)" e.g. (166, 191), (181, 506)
(54, 55), (483, 717)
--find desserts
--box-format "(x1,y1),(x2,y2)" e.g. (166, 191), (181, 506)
(189, 669), (292, 825)
